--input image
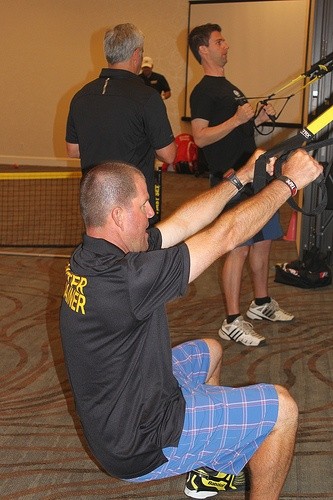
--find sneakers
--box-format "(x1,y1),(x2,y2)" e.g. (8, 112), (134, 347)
(217, 316), (266, 347)
(245, 297), (296, 322)
(182, 465), (252, 498)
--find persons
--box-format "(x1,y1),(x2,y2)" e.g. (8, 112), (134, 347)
(65, 22), (176, 230)
(137, 56), (172, 101)
(57, 149), (323, 500)
(188, 23), (295, 347)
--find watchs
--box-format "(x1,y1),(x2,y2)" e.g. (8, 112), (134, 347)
(223, 167), (244, 192)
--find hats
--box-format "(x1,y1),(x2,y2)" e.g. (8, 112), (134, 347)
(141, 56), (153, 68)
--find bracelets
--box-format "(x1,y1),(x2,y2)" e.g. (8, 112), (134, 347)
(277, 176), (298, 197)
